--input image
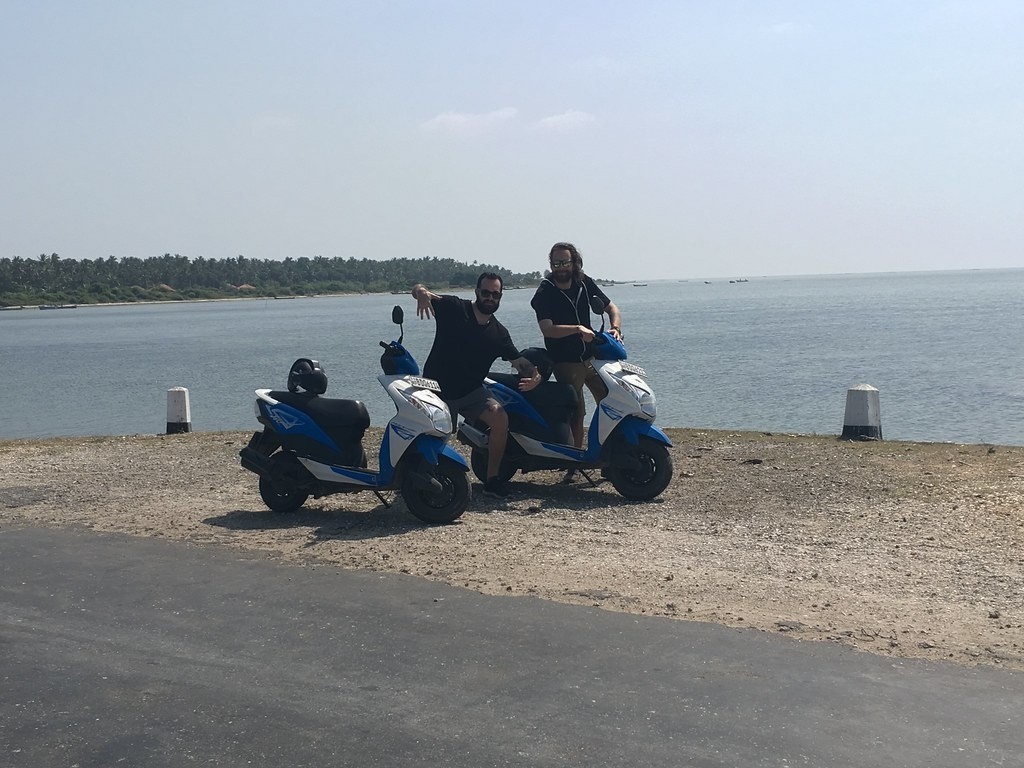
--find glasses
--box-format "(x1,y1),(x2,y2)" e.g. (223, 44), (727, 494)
(551, 260), (573, 268)
(478, 289), (503, 300)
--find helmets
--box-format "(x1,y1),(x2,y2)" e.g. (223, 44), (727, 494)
(518, 347), (554, 380)
(287, 358), (327, 394)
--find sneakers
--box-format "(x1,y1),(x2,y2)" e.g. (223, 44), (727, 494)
(482, 476), (510, 498)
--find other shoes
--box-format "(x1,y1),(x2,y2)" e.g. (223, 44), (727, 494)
(600, 468), (610, 477)
(564, 472), (581, 483)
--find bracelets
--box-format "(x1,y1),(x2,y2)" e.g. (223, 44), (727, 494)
(611, 326), (621, 334)
(576, 324), (580, 334)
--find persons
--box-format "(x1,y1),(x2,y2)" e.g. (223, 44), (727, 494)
(412, 273), (541, 498)
(531, 242), (625, 484)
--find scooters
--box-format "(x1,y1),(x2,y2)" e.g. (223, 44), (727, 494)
(239, 303), (471, 525)
(456, 294), (674, 500)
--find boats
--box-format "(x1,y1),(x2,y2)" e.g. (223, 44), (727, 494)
(503, 286), (514, 290)
(38, 304), (78, 309)
(633, 283), (648, 286)
(736, 278), (748, 282)
(704, 282), (712, 284)
(391, 291), (411, 294)
(729, 280), (736, 283)
(514, 286), (526, 289)
(602, 283), (614, 287)
(274, 296), (296, 299)
(0, 306), (23, 310)
